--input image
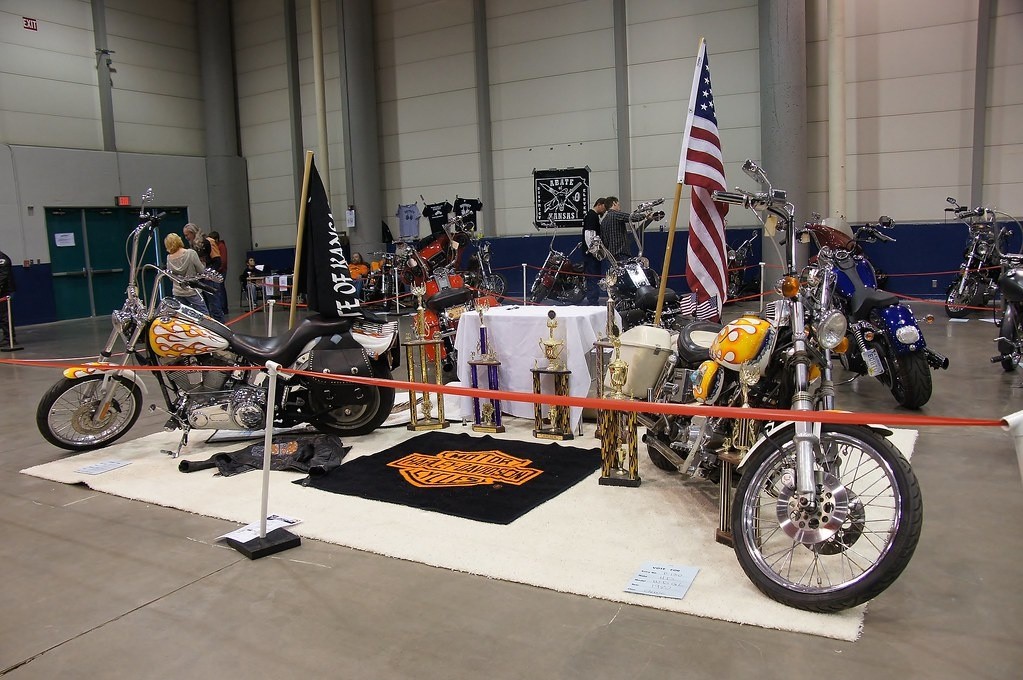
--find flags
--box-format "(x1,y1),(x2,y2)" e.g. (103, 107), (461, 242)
(680, 292), (697, 316)
(676, 45), (730, 322)
(301, 159), (386, 323)
(696, 296), (719, 320)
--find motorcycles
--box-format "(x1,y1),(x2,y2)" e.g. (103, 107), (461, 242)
(355, 188), (1023, 409)
(453, 235), (507, 304)
(36, 190), (398, 451)
(595, 158), (922, 614)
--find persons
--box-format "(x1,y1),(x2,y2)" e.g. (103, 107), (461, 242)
(600, 197), (653, 275)
(370, 258), (391, 284)
(0, 251), (16, 347)
(164, 223), (229, 323)
(241, 258), (271, 311)
(347, 252), (368, 297)
(582, 198), (608, 306)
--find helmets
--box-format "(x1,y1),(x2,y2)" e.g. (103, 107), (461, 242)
(822, 217), (854, 239)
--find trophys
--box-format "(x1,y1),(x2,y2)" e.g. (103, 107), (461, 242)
(593, 270), (629, 443)
(467, 298), (506, 434)
(530, 310), (573, 440)
(400, 284), (451, 430)
(599, 336), (641, 486)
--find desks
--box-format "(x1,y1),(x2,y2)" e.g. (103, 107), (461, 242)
(247, 274), (299, 309)
(453, 305), (623, 438)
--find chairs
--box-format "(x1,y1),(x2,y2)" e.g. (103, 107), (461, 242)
(239, 275), (267, 312)
(346, 260), (372, 304)
